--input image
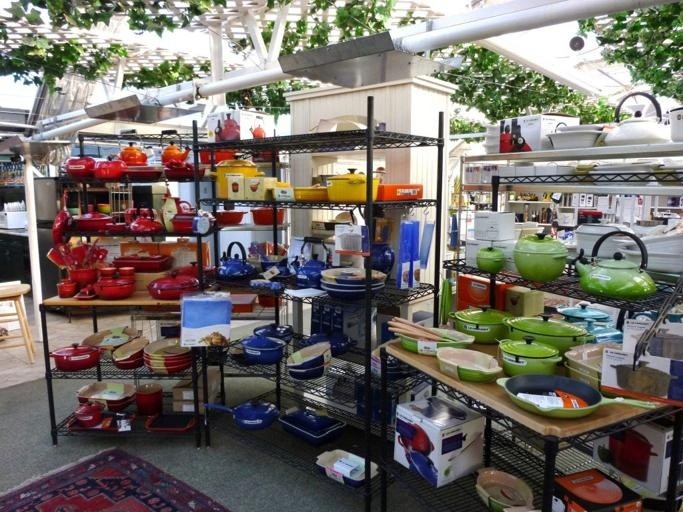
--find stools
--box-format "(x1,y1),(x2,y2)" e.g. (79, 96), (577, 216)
(0, 283), (37, 364)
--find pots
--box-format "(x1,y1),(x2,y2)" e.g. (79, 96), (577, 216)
(475, 246), (507, 275)
(394, 302), (610, 512)
(48, 151), (381, 432)
(574, 218), (636, 259)
(609, 360), (679, 398)
(646, 328), (683, 360)
(609, 428), (659, 482)
(512, 233), (570, 284)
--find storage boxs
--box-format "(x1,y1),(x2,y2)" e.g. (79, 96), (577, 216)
(355, 367), (433, 424)
(465, 238), (493, 268)
(474, 209), (516, 242)
(452, 272), (546, 318)
(593, 318), (682, 496)
(552, 468), (643, 512)
(392, 396), (486, 488)
(498, 113), (580, 153)
(207, 109), (274, 143)
(492, 239), (518, 272)
(311, 299), (434, 352)
(325, 361), (365, 402)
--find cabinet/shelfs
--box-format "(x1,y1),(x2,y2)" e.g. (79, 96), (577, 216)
(192, 95), (444, 512)
(378, 142), (683, 512)
(0, 232), (32, 298)
(38, 131), (225, 451)
(502, 191), (564, 241)
(200, 159), (293, 384)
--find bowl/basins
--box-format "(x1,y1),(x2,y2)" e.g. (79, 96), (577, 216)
(545, 123), (604, 150)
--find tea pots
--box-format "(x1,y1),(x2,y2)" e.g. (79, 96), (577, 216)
(572, 230), (658, 299)
(603, 90), (672, 147)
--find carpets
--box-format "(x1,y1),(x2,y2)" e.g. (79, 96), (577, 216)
(0, 446), (230, 512)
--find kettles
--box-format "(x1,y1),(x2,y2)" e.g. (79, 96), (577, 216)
(249, 115), (267, 138)
(216, 112), (241, 141)
(117, 133), (153, 165)
(160, 130), (191, 169)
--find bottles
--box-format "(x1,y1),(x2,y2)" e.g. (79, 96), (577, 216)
(523, 204), (546, 223)
